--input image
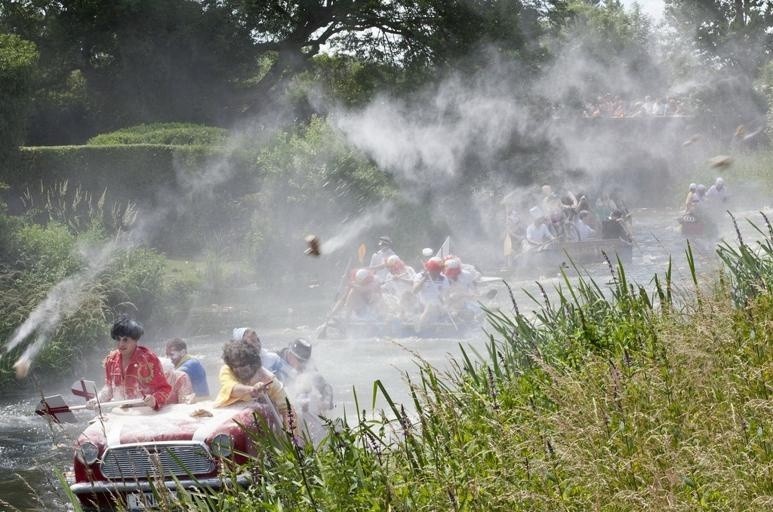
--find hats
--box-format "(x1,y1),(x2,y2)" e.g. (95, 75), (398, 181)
(528, 206), (546, 223)
(233, 327), (249, 343)
(689, 177), (724, 194)
(290, 338), (312, 361)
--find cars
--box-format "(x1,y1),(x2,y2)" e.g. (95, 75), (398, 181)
(68, 396), (290, 512)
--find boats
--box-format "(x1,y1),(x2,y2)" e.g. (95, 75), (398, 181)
(520, 239), (633, 272)
(331, 314), (468, 339)
(678, 213), (715, 235)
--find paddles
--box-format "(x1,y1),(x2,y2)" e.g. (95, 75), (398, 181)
(503, 205), (512, 256)
(314, 245), (367, 342)
(71, 377), (101, 398)
(34, 394), (148, 423)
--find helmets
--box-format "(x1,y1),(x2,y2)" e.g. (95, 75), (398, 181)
(423, 248), (462, 278)
(386, 255), (406, 276)
(355, 269), (373, 285)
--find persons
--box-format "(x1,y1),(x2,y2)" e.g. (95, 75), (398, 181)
(685, 177), (728, 213)
(85, 318), (333, 436)
(353, 235), (498, 334)
(505, 184), (633, 252)
